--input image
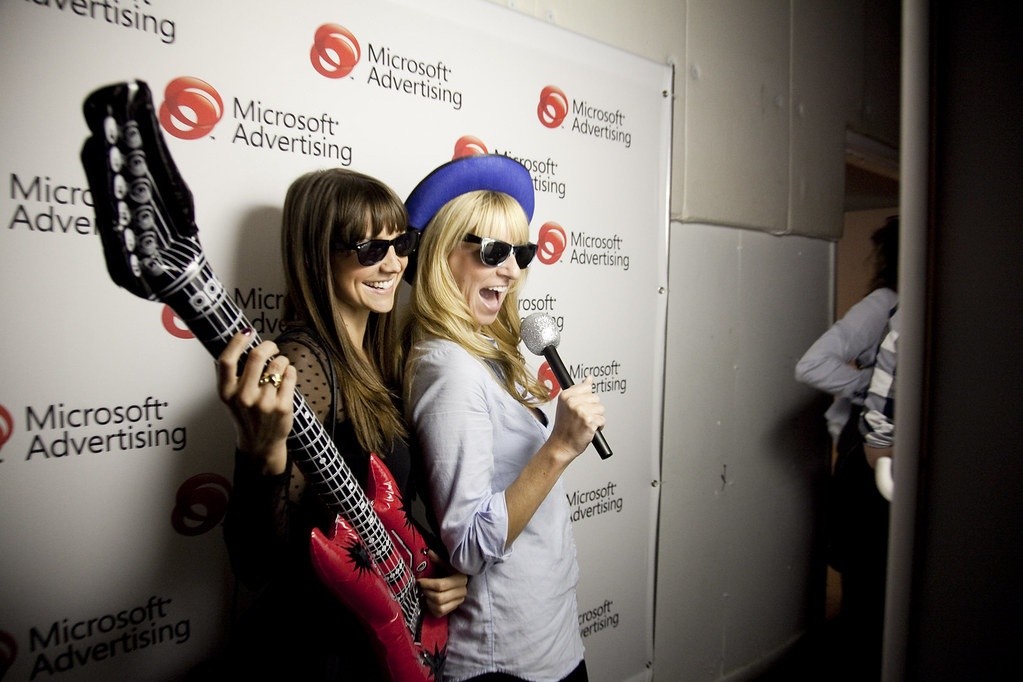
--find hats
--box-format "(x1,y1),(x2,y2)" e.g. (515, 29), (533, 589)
(403, 154), (534, 286)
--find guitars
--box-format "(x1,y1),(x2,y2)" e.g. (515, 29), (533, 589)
(78, 75), (452, 681)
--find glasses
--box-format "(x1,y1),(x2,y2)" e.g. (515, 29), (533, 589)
(463, 233), (539, 270)
(329, 227), (421, 266)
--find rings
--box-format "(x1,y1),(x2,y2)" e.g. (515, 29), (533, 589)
(260, 373), (281, 388)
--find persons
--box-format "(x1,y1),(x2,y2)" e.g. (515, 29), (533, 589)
(217, 168), (468, 682)
(794, 214), (899, 682)
(405, 154), (606, 682)
